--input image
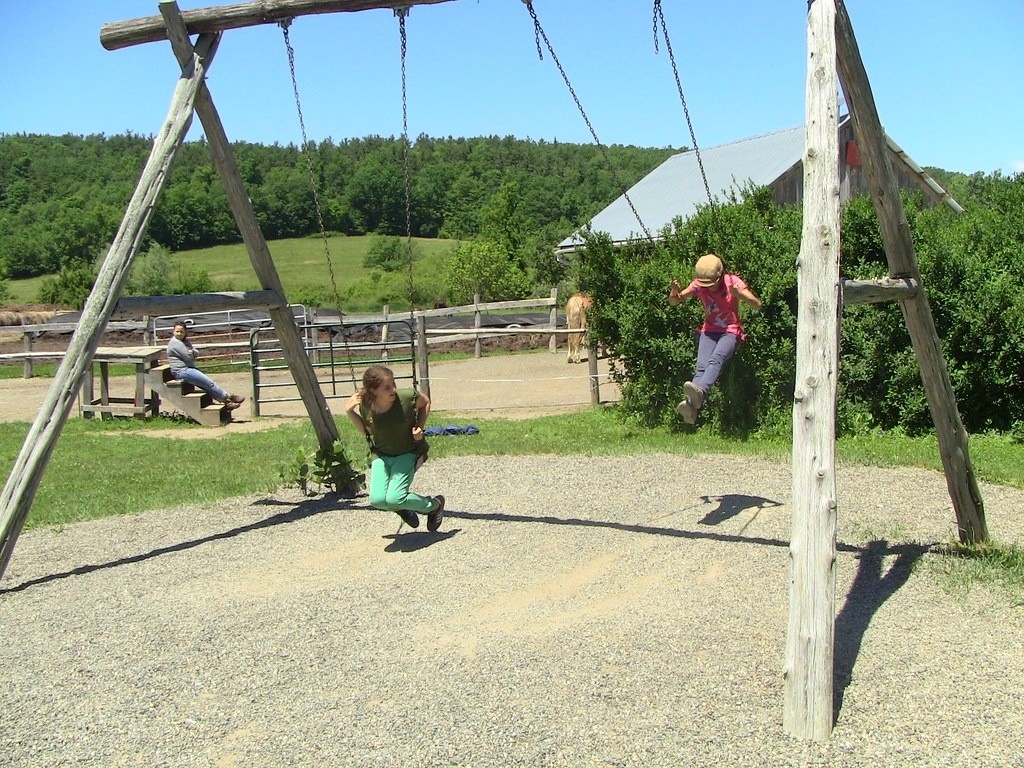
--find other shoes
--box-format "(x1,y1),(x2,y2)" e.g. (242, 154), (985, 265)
(678, 400), (697, 425)
(226, 400), (240, 410)
(230, 393), (245, 402)
(684, 381), (704, 409)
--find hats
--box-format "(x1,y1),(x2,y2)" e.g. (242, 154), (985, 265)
(695, 255), (723, 287)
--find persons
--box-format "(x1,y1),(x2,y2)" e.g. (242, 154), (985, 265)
(345, 366), (445, 532)
(166, 321), (245, 412)
(669, 254), (761, 424)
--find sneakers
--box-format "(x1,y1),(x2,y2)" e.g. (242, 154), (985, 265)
(427, 495), (445, 531)
(394, 510), (419, 528)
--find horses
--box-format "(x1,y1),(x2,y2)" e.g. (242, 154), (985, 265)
(566, 295), (610, 364)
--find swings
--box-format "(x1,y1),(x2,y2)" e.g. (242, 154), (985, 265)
(524, 0), (749, 350)
(278, 15), (426, 463)
